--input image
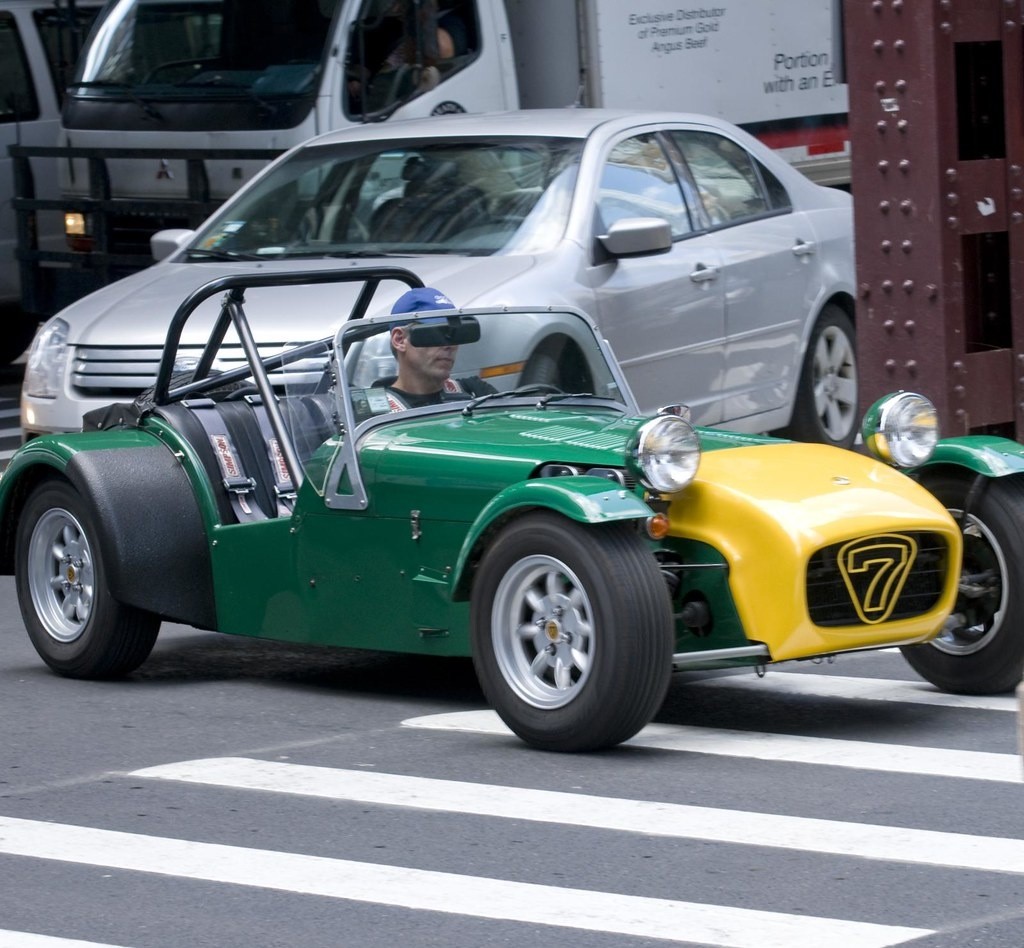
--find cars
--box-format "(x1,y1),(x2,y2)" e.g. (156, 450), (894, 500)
(19, 105), (861, 450)
(0, 0), (107, 305)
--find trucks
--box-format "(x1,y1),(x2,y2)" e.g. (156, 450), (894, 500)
(51, 0), (849, 287)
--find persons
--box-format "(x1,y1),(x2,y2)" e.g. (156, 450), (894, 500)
(361, 286), (501, 417)
(527, 139), (625, 230)
(348, 1), (456, 101)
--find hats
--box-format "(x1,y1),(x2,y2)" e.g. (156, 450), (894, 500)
(389, 287), (459, 333)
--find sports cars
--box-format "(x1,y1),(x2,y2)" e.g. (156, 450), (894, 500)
(0, 264), (1024, 756)
